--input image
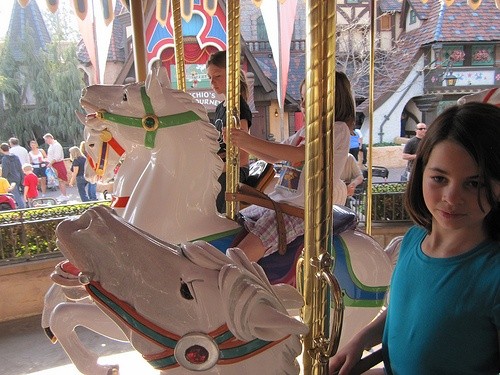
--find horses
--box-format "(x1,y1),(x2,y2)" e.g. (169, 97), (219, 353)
(41, 66), (406, 375)
(50, 204), (310, 375)
(456, 87), (500, 110)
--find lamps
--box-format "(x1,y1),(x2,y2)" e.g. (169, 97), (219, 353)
(444, 71), (458, 86)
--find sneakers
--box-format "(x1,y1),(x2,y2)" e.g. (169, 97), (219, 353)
(56, 195), (69, 202)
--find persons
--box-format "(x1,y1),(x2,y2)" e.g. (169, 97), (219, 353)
(0, 133), (101, 211)
(328, 102), (500, 375)
(221, 70), (357, 262)
(340, 152), (364, 209)
(349, 127), (368, 167)
(401, 122), (427, 180)
(203, 50), (252, 214)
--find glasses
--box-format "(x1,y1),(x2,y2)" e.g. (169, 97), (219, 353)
(417, 128), (426, 130)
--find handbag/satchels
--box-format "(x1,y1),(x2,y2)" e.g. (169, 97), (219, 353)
(45, 166), (60, 189)
